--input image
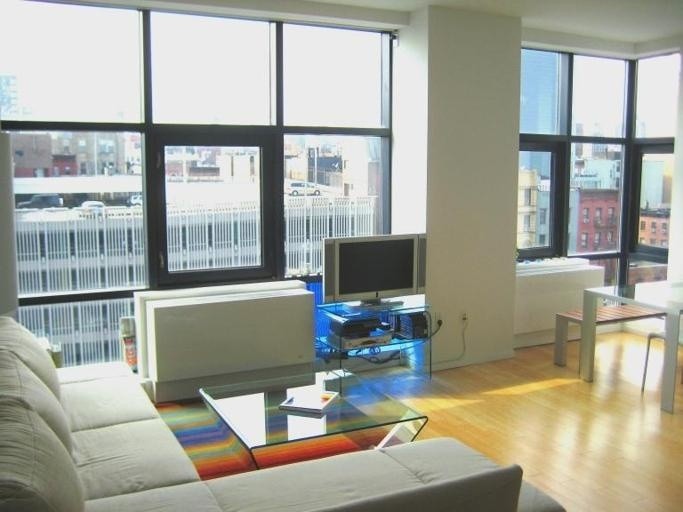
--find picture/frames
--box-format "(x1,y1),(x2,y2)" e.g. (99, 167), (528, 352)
(315, 299), (433, 395)
(578, 278), (683, 414)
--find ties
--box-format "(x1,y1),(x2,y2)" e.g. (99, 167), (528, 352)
(323, 233), (427, 310)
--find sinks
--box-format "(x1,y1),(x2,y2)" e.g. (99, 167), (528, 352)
(0, 315), (569, 512)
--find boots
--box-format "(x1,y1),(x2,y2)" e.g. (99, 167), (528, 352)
(17, 194), (64, 209)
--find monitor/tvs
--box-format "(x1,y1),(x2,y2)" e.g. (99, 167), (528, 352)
(278, 389), (339, 415)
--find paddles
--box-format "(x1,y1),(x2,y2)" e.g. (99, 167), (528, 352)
(148, 380), (448, 481)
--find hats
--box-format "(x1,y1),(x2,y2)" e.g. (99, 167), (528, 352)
(126, 195), (142, 206)
(286, 181), (322, 196)
(72, 201), (106, 216)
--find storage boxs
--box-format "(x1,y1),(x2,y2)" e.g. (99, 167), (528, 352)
(550, 302), (664, 368)
